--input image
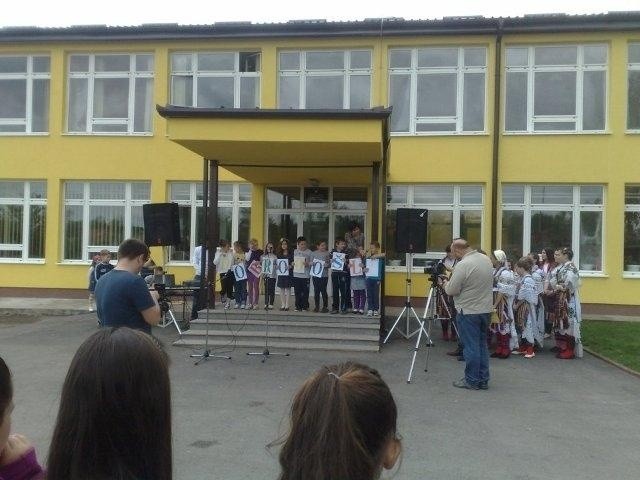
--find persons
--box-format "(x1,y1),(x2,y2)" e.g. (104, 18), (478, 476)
(191, 222), (385, 319)
(0, 357), (46, 480)
(49, 326), (172, 479)
(88, 249), (162, 313)
(95, 238), (162, 334)
(266, 361), (404, 479)
(437, 238), (583, 390)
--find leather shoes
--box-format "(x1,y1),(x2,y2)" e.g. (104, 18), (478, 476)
(446, 345), (462, 355)
(490, 346), (535, 359)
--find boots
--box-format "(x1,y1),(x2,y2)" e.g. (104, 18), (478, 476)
(551, 331), (575, 358)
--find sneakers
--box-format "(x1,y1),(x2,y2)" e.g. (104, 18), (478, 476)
(223, 302), (289, 311)
(294, 307), (379, 316)
(453, 378), (488, 390)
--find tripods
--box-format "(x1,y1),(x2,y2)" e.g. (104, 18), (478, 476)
(247, 287), (289, 363)
(384, 263), (435, 346)
(155, 297), (182, 336)
(406, 292), (466, 384)
(190, 295), (232, 364)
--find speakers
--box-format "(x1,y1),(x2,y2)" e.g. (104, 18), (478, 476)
(395, 207), (428, 254)
(143, 202), (180, 246)
